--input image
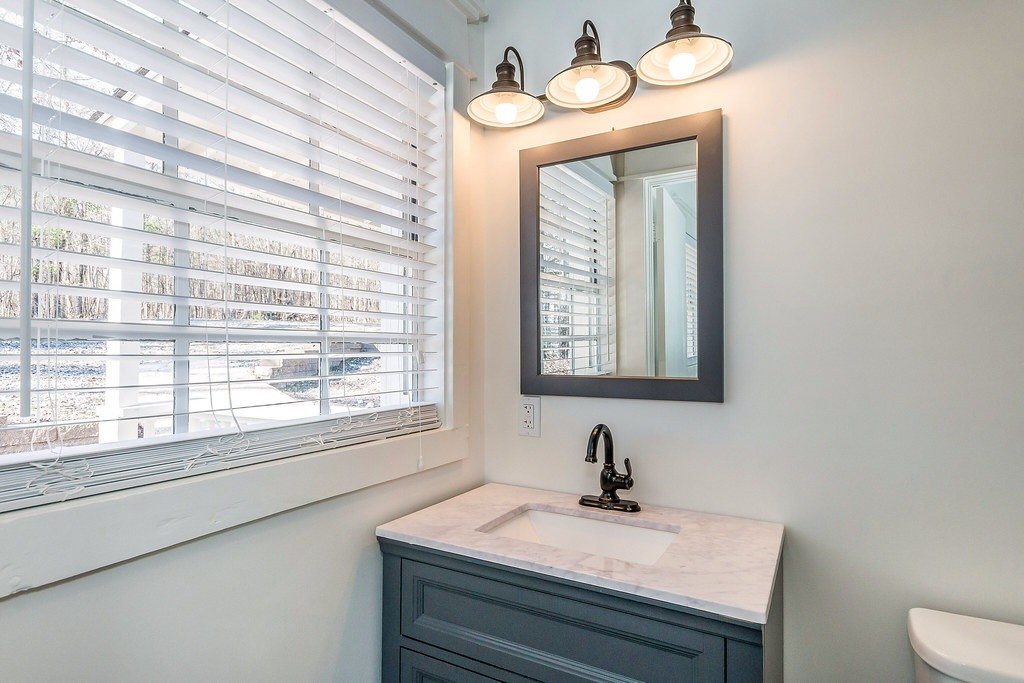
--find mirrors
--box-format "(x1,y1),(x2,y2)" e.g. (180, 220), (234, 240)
(520, 109), (726, 403)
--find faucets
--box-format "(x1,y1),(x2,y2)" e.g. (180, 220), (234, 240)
(578, 422), (641, 512)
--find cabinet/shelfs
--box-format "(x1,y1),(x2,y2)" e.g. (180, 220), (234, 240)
(373, 537), (785, 683)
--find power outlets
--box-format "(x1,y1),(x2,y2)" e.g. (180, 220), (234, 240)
(518, 396), (541, 438)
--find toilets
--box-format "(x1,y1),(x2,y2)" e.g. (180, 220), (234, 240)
(908, 606), (1024, 682)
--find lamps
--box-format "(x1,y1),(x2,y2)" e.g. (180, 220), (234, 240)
(468, 1), (735, 129)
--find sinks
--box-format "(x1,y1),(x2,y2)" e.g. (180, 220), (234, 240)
(477, 501), (684, 565)
